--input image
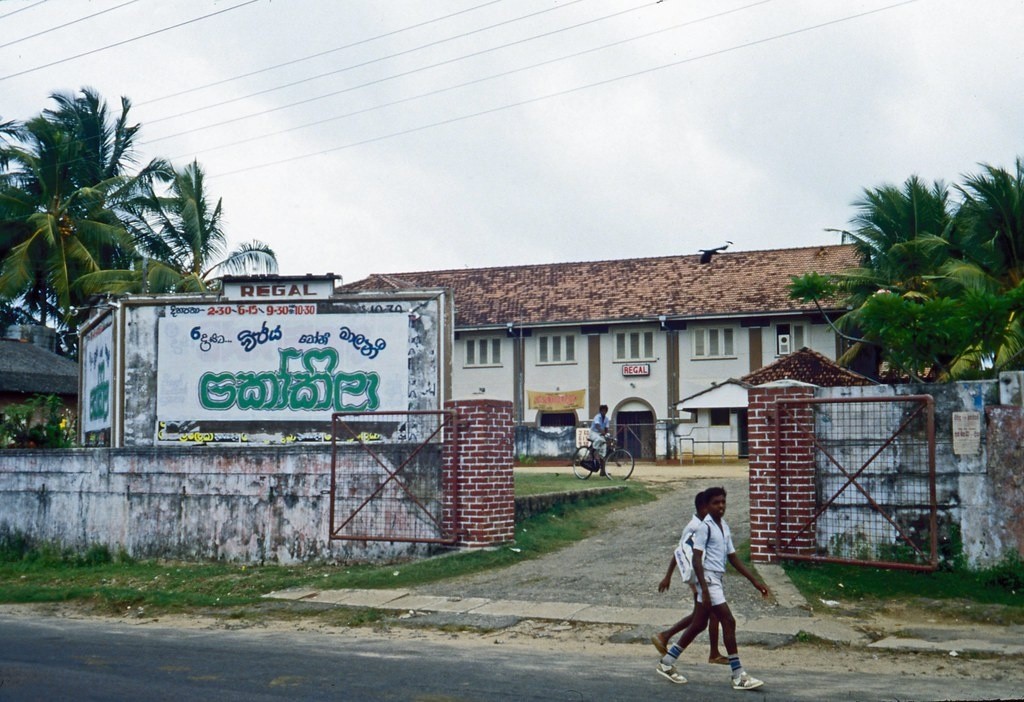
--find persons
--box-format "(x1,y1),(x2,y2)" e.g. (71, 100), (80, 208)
(651, 492), (731, 665)
(589, 405), (615, 476)
(657, 486), (769, 688)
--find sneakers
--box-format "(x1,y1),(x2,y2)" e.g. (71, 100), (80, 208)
(709, 655), (730, 665)
(656, 659), (687, 683)
(651, 634), (668, 657)
(731, 672), (764, 691)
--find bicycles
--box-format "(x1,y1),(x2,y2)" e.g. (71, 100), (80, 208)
(571, 435), (636, 481)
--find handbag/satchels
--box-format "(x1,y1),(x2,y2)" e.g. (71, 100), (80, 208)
(673, 521), (711, 585)
